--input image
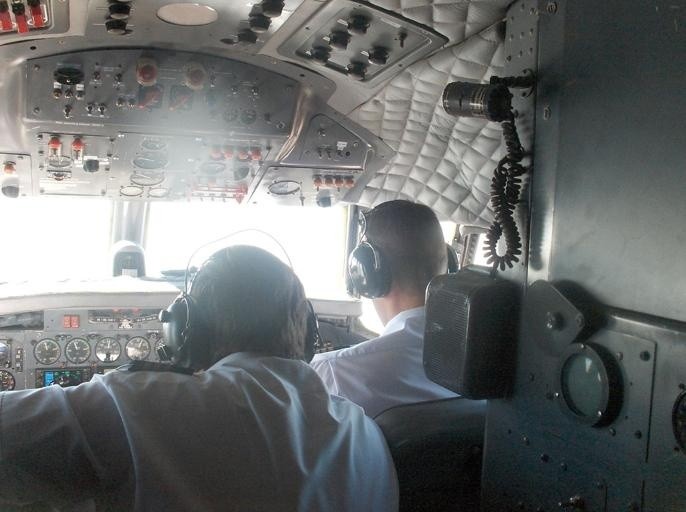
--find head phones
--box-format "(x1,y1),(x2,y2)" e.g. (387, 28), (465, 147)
(346, 199), (459, 299)
(156, 225), (324, 370)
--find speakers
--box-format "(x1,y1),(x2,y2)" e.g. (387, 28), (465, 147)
(422, 270), (517, 401)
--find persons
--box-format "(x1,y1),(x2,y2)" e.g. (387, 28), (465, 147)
(0, 244), (401, 512)
(309, 197), (458, 417)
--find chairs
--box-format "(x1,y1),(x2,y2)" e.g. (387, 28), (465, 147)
(368, 397), (485, 510)
